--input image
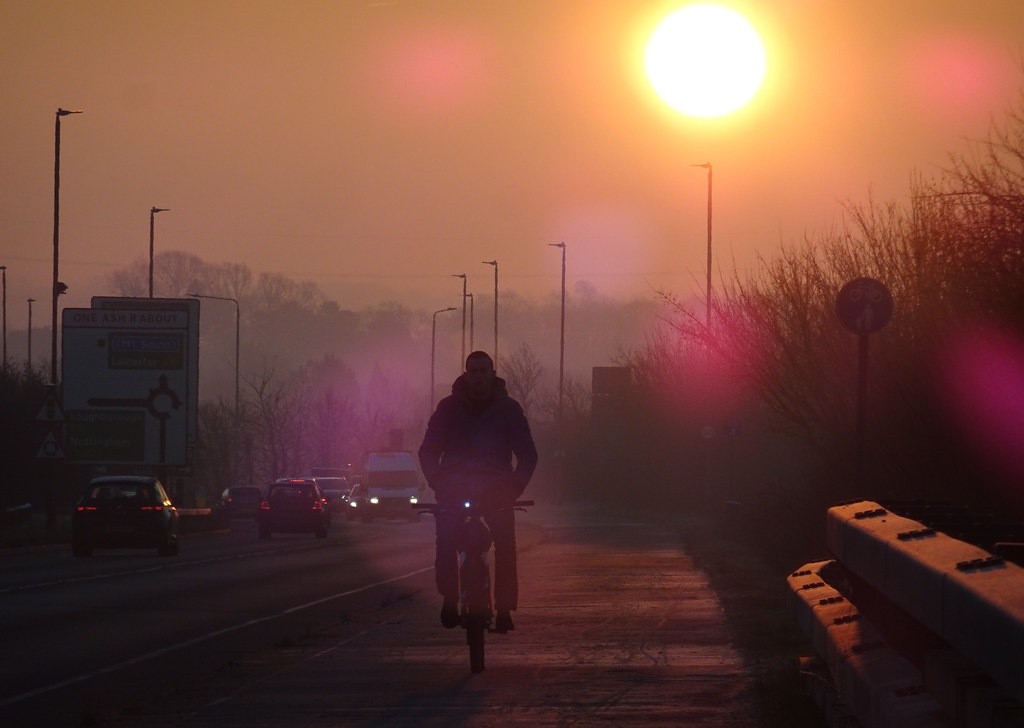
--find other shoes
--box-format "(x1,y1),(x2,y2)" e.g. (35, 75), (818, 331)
(496, 610), (515, 632)
(441, 593), (459, 628)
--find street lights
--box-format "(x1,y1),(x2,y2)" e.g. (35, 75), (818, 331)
(548, 241), (564, 414)
(459, 293), (474, 353)
(690, 161), (716, 366)
(452, 273), (468, 376)
(48, 107), (83, 378)
(147, 206), (169, 299)
(27, 296), (37, 372)
(481, 261), (498, 371)
(1, 265), (8, 373)
(188, 293), (241, 419)
(428, 307), (458, 416)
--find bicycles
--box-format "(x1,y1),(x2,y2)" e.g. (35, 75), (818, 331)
(408, 499), (536, 676)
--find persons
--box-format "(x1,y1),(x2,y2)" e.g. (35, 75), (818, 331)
(418, 351), (538, 635)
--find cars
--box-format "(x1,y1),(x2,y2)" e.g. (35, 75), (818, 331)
(73, 475), (184, 557)
(345, 483), (367, 520)
(314, 474), (352, 512)
(261, 475), (333, 543)
(218, 483), (267, 524)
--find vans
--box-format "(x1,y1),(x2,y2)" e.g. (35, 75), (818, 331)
(364, 451), (428, 523)
